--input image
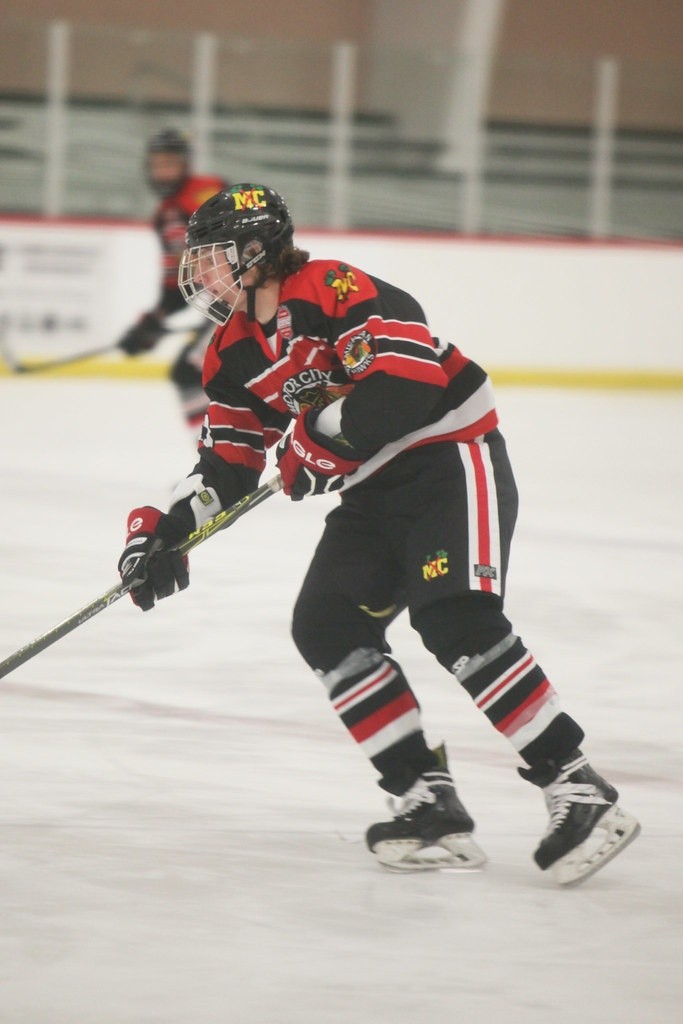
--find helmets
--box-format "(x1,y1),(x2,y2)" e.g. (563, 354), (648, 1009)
(178, 183), (309, 327)
(141, 128), (193, 199)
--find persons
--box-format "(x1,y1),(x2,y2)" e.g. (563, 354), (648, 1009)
(117, 126), (235, 439)
(118, 179), (642, 887)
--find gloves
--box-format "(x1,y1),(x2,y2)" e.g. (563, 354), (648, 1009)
(276, 407), (361, 501)
(118, 312), (170, 357)
(118, 507), (190, 611)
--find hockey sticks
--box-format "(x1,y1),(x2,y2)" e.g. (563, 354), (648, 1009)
(17, 317), (207, 372)
(2, 475), (285, 676)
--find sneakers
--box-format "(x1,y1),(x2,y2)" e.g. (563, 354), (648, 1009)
(518, 749), (640, 886)
(366, 743), (485, 869)
